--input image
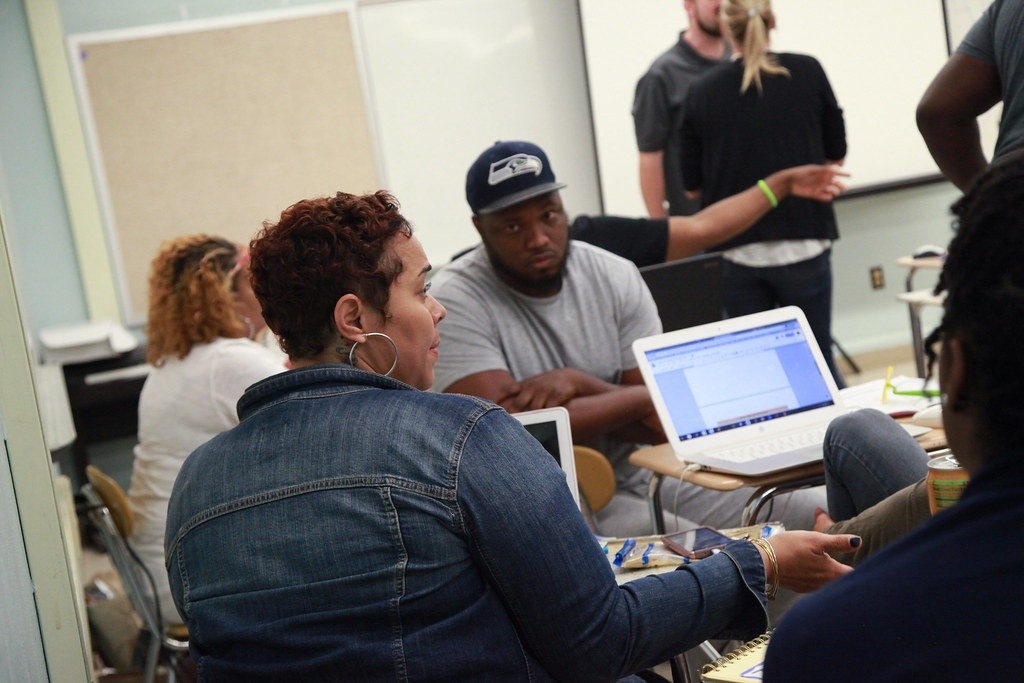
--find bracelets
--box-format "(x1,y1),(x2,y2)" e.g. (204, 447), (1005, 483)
(755, 536), (780, 601)
(750, 178), (780, 207)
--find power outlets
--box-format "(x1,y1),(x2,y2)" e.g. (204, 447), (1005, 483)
(868, 266), (886, 290)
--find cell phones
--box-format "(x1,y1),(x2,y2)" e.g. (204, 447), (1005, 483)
(659, 525), (735, 558)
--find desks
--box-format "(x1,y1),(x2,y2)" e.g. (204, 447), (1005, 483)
(900, 255), (944, 379)
(627, 376), (947, 683)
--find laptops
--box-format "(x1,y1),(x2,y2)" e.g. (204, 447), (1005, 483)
(507, 407), (580, 512)
(632, 305), (932, 476)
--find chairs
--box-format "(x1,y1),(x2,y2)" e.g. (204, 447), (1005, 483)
(82, 464), (189, 683)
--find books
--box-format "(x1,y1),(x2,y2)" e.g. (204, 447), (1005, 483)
(701, 627), (777, 683)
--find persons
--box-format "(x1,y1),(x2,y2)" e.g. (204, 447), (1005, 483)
(128, 0), (848, 656)
(761, 175), (1024, 683)
(154, 191), (861, 683)
(917, 1), (1023, 196)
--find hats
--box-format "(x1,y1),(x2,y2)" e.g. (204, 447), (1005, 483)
(465, 139), (567, 215)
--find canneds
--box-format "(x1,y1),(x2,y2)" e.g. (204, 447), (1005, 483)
(926, 454), (970, 516)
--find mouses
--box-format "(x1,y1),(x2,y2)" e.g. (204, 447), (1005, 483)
(912, 244), (946, 259)
